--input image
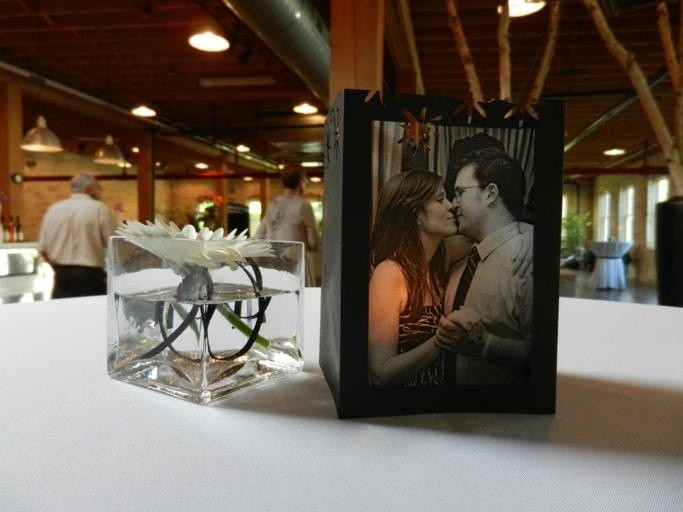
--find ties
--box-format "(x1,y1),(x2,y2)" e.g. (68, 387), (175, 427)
(444, 248), (479, 387)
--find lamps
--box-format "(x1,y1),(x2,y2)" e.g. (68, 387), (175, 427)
(187, 22), (230, 53)
(92, 86), (127, 165)
(20, 74), (62, 153)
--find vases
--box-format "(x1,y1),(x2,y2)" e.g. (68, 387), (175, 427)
(107, 236), (304, 404)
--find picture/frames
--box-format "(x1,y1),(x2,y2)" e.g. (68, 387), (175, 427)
(319, 89), (562, 420)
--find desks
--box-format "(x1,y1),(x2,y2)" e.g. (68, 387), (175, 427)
(587, 241), (634, 290)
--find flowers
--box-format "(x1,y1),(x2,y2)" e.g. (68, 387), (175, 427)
(114, 219), (276, 360)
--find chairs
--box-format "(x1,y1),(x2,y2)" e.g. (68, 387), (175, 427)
(27, 262), (55, 300)
(18, 293), (33, 304)
(0, 286), (683, 511)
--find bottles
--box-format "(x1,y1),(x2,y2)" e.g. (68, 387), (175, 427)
(15, 216), (23, 241)
(7, 216), (15, 242)
(0, 216), (7, 243)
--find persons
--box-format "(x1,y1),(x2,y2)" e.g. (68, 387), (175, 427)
(434, 148), (535, 391)
(38, 174), (122, 298)
(253, 162), (325, 287)
(368, 170), (490, 389)
